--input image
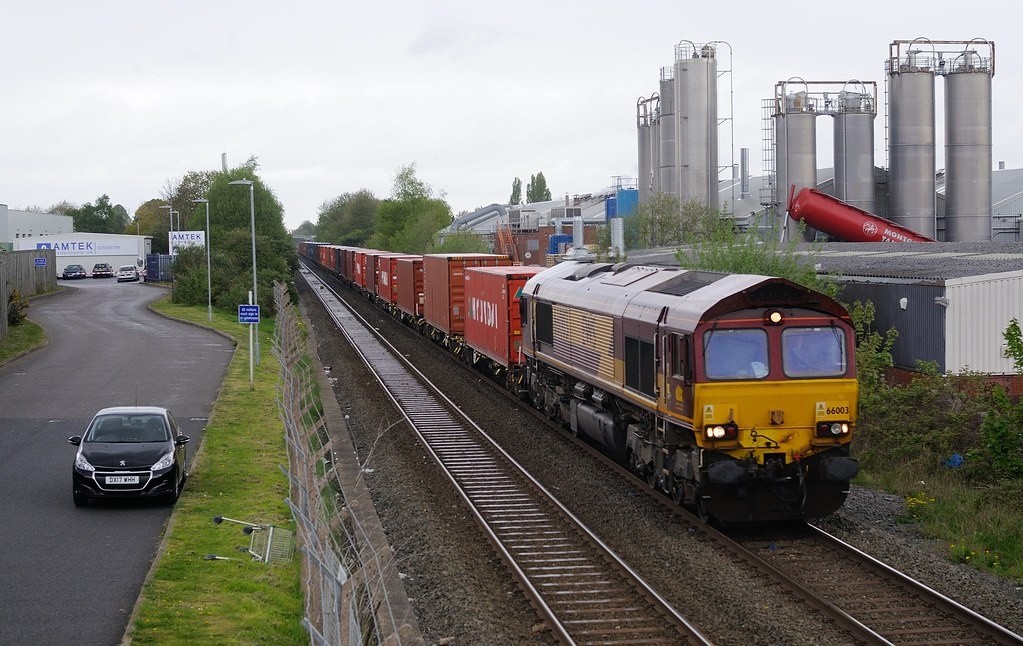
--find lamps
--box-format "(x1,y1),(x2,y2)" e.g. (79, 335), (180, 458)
(934, 297), (950, 309)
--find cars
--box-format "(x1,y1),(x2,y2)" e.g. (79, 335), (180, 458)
(63, 265), (87, 280)
(92, 262), (114, 278)
(68, 405), (191, 507)
(115, 264), (140, 282)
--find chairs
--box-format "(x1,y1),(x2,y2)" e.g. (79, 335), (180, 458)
(97, 418), (161, 441)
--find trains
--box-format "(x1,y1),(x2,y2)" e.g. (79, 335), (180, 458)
(299, 240), (867, 525)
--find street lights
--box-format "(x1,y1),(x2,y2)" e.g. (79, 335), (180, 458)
(192, 199), (212, 322)
(159, 204), (175, 303)
(168, 211), (180, 245)
(227, 178), (260, 366)
